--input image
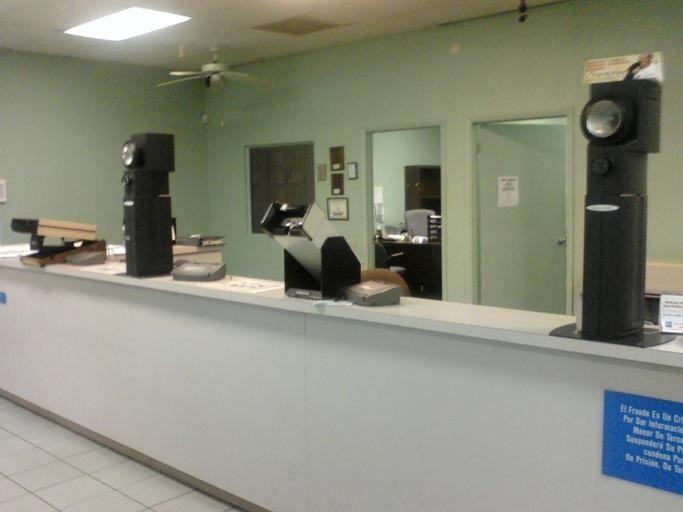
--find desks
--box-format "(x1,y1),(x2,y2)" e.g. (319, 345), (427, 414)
(375, 239), (442, 292)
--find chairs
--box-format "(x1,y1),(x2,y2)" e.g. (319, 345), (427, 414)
(404, 208), (437, 239)
(374, 241), (420, 292)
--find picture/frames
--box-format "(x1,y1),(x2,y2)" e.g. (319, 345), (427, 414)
(347, 162), (358, 180)
(326, 197), (350, 222)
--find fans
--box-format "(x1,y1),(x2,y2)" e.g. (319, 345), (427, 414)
(153, 43), (271, 93)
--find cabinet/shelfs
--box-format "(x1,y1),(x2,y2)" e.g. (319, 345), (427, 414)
(427, 215), (441, 242)
(404, 165), (441, 215)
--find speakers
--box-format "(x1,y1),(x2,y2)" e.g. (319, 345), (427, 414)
(121, 133), (175, 277)
(580, 80), (661, 340)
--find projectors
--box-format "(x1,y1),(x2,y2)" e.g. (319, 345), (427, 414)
(258, 199), (338, 289)
